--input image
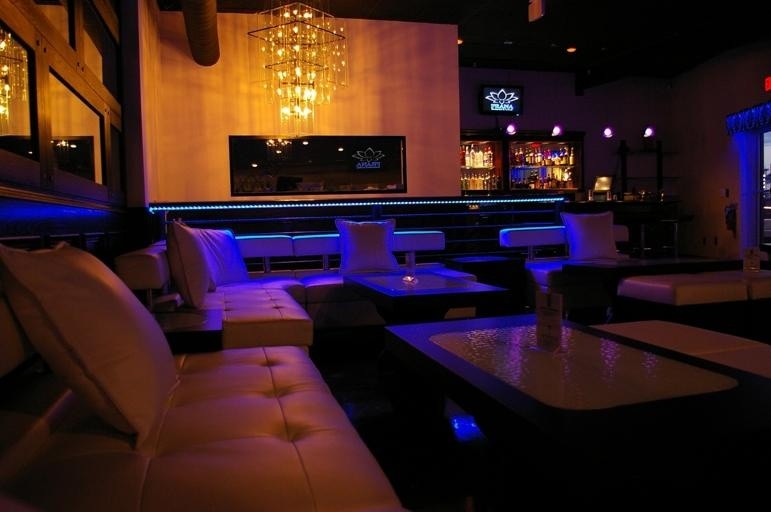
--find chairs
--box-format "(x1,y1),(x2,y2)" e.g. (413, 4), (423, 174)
(659, 207), (698, 257)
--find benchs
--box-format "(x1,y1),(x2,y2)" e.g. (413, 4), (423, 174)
(497, 224), (630, 319)
(115, 235), (313, 356)
(293, 231), (478, 327)
(0, 293), (411, 512)
(617, 269), (771, 319)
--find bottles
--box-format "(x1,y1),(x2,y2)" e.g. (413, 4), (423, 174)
(511, 147), (576, 189)
(458, 143), (501, 190)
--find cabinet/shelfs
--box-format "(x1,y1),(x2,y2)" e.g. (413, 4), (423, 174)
(459, 126), (587, 193)
(615, 140), (682, 192)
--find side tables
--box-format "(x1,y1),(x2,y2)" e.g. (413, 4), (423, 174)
(150, 307), (223, 355)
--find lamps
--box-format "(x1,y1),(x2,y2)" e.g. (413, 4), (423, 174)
(265, 140), (292, 160)
(0, 28), (28, 120)
(246, 0), (346, 135)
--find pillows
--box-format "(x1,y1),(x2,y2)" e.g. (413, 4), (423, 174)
(558, 211), (617, 262)
(334, 217), (401, 273)
(0, 241), (183, 451)
(166, 218), (249, 309)
